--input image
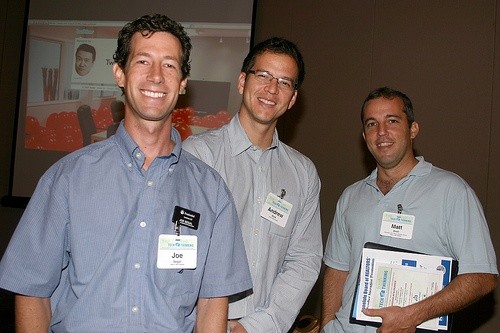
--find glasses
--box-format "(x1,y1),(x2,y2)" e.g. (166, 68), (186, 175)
(248, 70), (298, 90)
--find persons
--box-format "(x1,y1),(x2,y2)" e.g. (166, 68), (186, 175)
(75, 44), (96, 77)
(0, 14), (253, 333)
(319, 87), (498, 333)
(182, 37), (324, 333)
